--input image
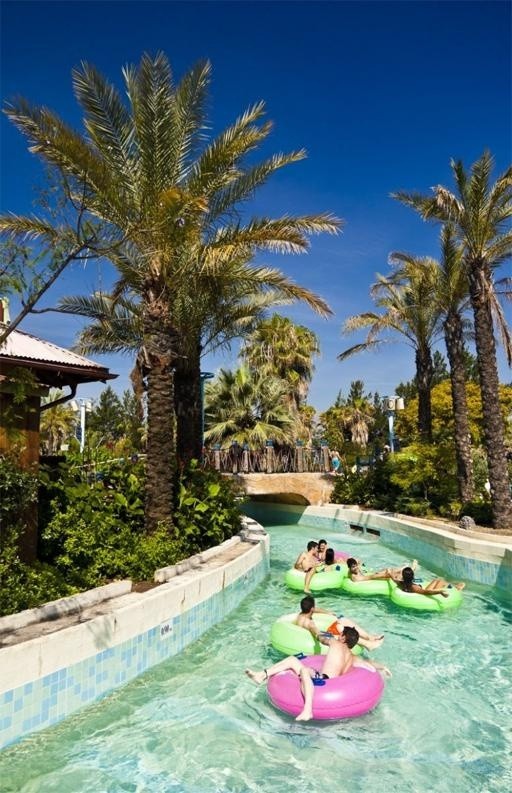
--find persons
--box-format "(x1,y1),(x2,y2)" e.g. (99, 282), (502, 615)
(329, 446), (344, 472)
(296, 595), (384, 650)
(245, 626), (394, 722)
(294, 538), (466, 598)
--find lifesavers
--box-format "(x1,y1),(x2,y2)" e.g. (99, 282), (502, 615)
(266, 653), (384, 719)
(286, 550), (463, 611)
(270, 613), (363, 657)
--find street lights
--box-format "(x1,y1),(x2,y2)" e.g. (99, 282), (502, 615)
(382, 393), (405, 452)
(68, 395), (94, 455)
